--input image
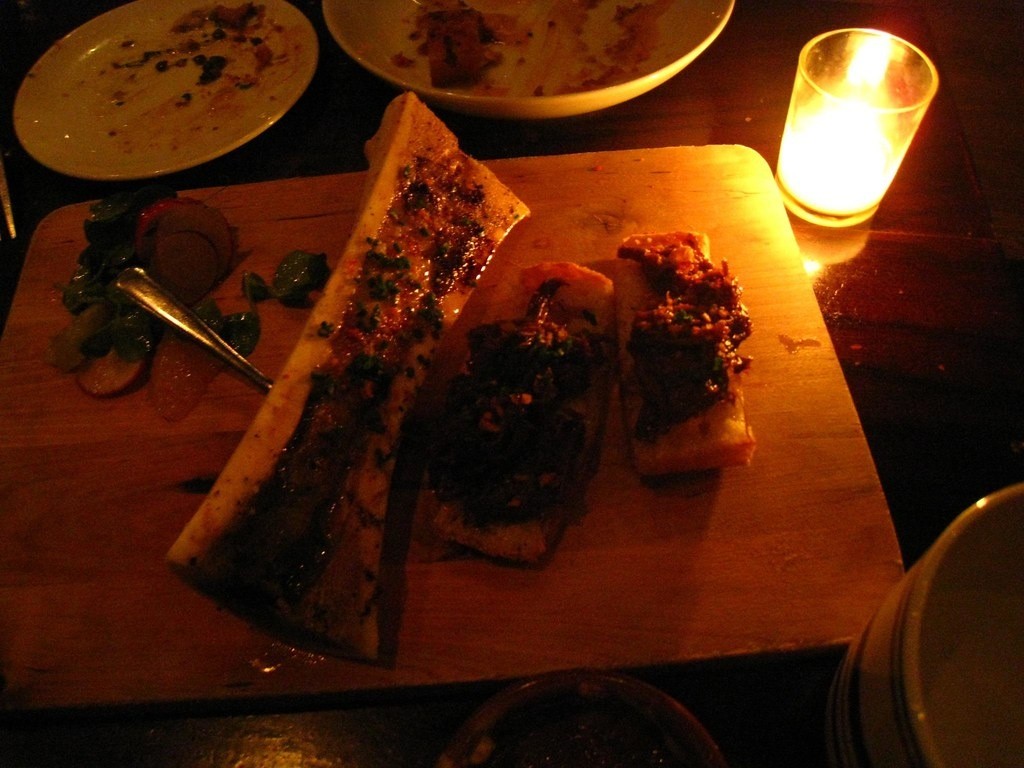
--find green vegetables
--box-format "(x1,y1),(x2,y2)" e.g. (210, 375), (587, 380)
(53, 192), (332, 362)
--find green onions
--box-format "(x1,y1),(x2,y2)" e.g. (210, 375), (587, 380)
(309, 165), (724, 526)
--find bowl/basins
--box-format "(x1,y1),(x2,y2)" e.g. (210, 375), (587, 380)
(824, 483), (1024, 768)
(433, 672), (728, 768)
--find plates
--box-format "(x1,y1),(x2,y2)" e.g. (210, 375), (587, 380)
(13, 0), (320, 180)
(321, 0), (736, 120)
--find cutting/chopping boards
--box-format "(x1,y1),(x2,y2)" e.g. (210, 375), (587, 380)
(0, 144), (904, 717)
